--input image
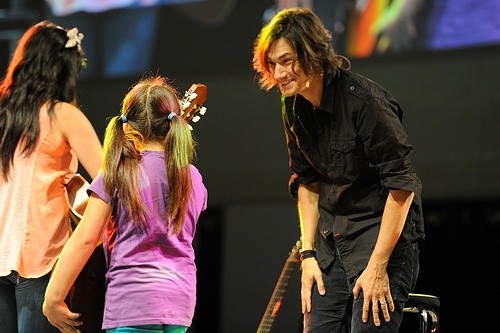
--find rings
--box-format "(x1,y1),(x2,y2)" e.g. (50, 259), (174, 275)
(380, 300), (387, 305)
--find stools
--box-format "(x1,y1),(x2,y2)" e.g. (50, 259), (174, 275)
(403, 293), (440, 333)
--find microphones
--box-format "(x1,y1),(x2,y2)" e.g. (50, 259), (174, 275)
(305, 73), (316, 88)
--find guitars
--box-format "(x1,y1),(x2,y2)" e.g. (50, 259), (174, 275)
(66, 83), (208, 248)
(256, 235), (303, 333)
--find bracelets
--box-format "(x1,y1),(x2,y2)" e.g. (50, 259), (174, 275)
(300, 250), (316, 261)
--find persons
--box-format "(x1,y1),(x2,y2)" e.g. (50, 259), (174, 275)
(0, 20), (103, 333)
(43, 77), (208, 333)
(253, 7), (425, 333)
